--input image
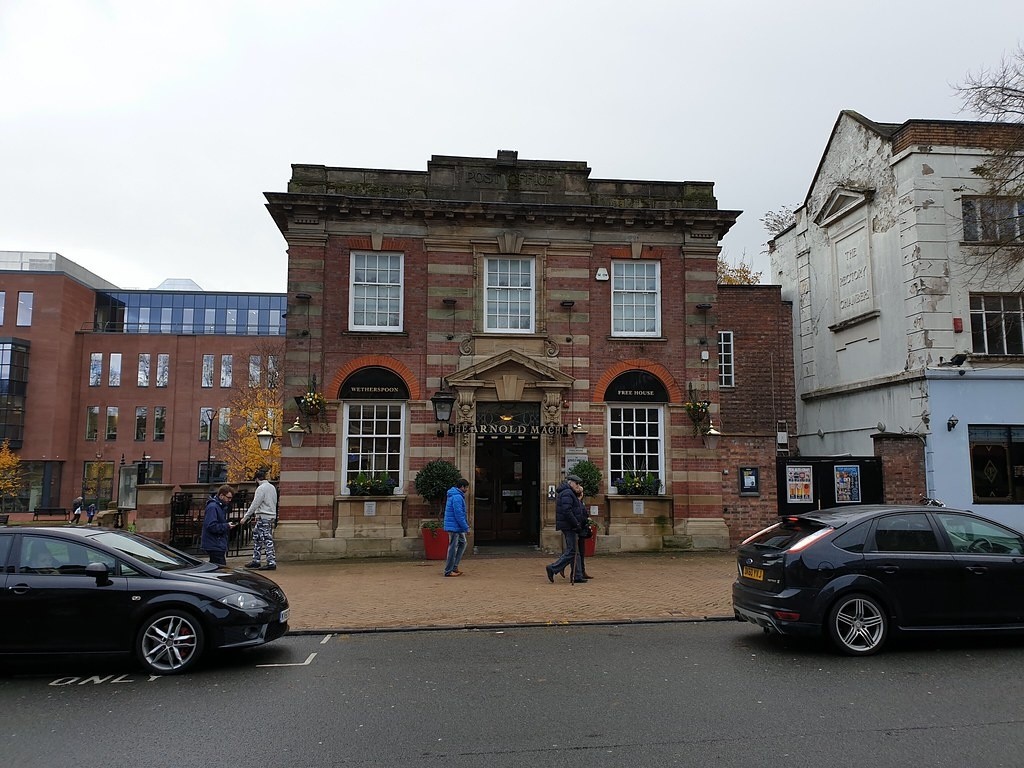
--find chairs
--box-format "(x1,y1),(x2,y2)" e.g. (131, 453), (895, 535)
(173, 515), (205, 550)
(28, 537), (61, 566)
(67, 544), (90, 566)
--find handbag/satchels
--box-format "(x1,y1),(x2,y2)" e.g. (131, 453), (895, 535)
(74, 508), (82, 514)
(585, 525), (593, 539)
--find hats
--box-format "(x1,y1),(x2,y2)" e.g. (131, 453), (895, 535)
(253, 472), (265, 480)
(567, 475), (582, 483)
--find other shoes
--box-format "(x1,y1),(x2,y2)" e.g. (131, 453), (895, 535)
(571, 578), (587, 583)
(545, 566), (555, 583)
(583, 573), (594, 579)
(446, 571), (460, 576)
(245, 561), (261, 568)
(560, 569), (565, 578)
(259, 563), (276, 570)
(454, 569), (463, 575)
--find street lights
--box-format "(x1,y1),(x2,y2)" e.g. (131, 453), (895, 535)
(206, 408), (218, 483)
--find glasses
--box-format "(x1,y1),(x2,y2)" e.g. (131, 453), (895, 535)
(223, 493), (232, 500)
(570, 480), (580, 485)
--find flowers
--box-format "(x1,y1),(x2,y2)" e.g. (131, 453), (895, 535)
(345, 471), (396, 496)
(687, 382), (710, 438)
(296, 377), (330, 436)
(613, 470), (664, 495)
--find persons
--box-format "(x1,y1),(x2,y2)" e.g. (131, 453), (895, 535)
(86, 504), (96, 525)
(240, 472), (277, 571)
(68, 496), (83, 526)
(443, 479), (470, 576)
(200, 484), (240, 566)
(547, 474), (594, 583)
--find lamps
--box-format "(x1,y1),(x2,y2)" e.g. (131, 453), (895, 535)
(950, 353), (967, 366)
(296, 293), (312, 299)
(443, 298), (457, 304)
(702, 419), (721, 450)
(428, 374), (456, 437)
(560, 300), (574, 306)
(696, 303), (712, 309)
(947, 415), (959, 431)
(287, 416), (306, 447)
(570, 416), (588, 447)
(257, 420), (283, 450)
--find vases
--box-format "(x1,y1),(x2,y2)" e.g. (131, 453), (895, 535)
(300, 402), (322, 415)
(349, 488), (394, 496)
(689, 410), (706, 424)
(618, 489), (659, 495)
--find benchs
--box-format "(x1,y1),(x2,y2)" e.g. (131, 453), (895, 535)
(33, 507), (71, 521)
(0, 514), (10, 524)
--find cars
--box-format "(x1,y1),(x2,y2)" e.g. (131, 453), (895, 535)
(731, 504), (1023, 658)
(0, 524), (291, 677)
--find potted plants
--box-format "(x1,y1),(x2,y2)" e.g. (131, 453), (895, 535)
(565, 457), (601, 558)
(413, 457), (464, 561)
